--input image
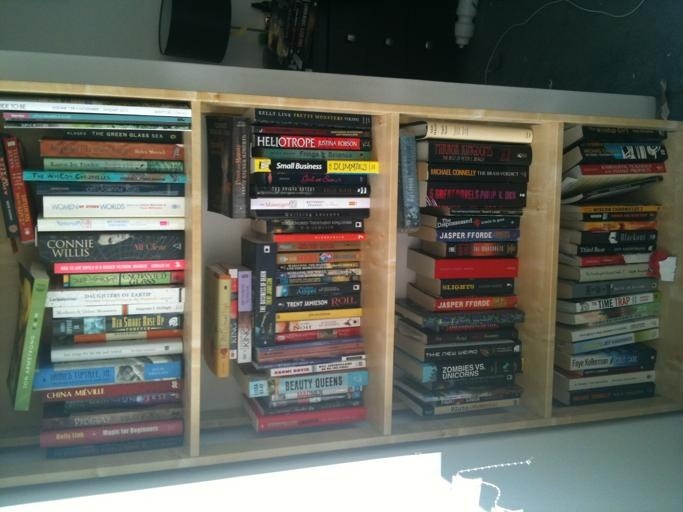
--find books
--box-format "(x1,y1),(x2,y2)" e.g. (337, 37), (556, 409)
(0, 96), (193, 459)
(553, 125), (669, 405)
(201, 106), (380, 436)
(393, 118), (535, 418)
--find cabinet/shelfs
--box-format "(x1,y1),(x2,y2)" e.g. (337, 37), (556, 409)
(0, 79), (683, 490)
